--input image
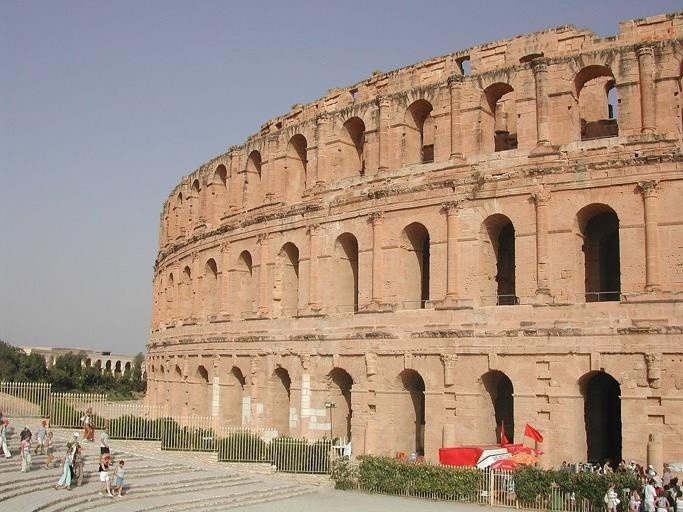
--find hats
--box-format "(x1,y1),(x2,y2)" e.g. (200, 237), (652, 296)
(73, 433), (80, 437)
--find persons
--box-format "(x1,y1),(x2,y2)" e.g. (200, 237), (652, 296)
(0, 406), (127, 499)
(553, 457), (682, 511)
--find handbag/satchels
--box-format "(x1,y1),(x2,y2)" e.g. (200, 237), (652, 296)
(604, 493), (609, 504)
(629, 496), (640, 512)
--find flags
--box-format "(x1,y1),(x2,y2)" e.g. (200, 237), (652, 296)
(500, 423), (508, 444)
(523, 423), (543, 443)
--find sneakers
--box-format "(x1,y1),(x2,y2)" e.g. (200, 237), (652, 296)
(99, 489), (124, 499)
